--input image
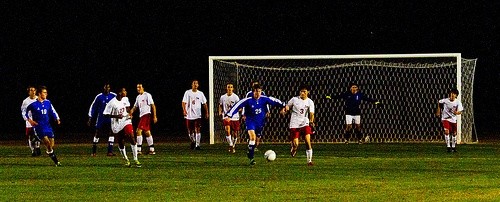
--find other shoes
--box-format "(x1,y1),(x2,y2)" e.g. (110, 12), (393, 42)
(191, 138), (196, 149)
(36, 146), (41, 155)
(447, 147), (452, 153)
(452, 146), (455, 153)
(196, 145), (201, 151)
(31, 148), (36, 157)
(358, 140), (362, 144)
(91, 152), (96, 157)
(107, 152), (113, 156)
(345, 140), (349, 144)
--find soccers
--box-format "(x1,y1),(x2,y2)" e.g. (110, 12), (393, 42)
(264, 150), (276, 161)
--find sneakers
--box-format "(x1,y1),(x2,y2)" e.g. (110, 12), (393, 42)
(122, 157), (130, 167)
(137, 147), (142, 155)
(228, 146), (235, 153)
(290, 146), (297, 158)
(149, 146), (156, 155)
(250, 159), (256, 165)
(254, 147), (259, 152)
(56, 161), (62, 167)
(246, 144), (249, 155)
(307, 162), (314, 166)
(133, 160), (142, 167)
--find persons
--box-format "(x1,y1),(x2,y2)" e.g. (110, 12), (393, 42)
(25, 86), (61, 166)
(20, 86), (47, 158)
(326, 85), (379, 145)
(181, 79), (209, 151)
(219, 82), (240, 154)
(223, 85), (289, 166)
(88, 82), (119, 158)
(281, 86), (315, 166)
(129, 83), (157, 154)
(241, 80), (270, 154)
(102, 86), (142, 167)
(436, 88), (463, 148)
